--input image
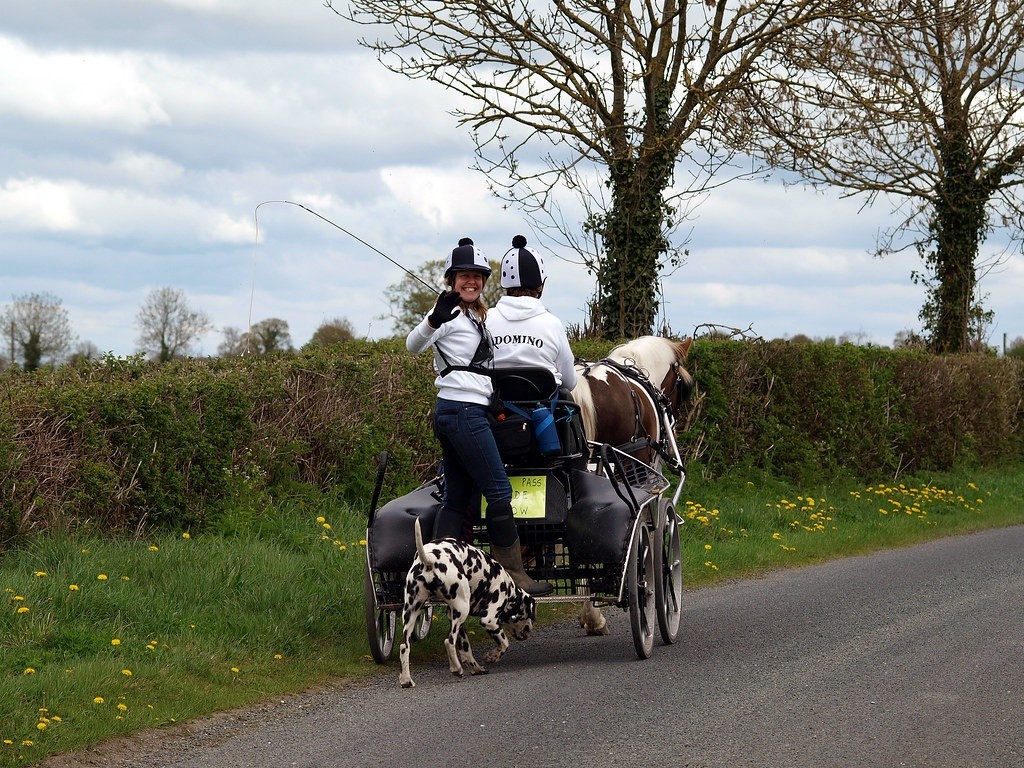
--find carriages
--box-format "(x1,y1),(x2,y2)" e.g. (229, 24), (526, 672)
(364, 327), (695, 665)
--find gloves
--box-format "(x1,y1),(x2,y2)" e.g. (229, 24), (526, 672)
(428, 290), (463, 328)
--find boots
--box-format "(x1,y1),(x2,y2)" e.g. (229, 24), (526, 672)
(490, 537), (555, 596)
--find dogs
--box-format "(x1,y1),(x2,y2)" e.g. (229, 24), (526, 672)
(399, 516), (540, 689)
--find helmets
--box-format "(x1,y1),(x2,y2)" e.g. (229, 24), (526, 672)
(500, 235), (547, 288)
(444, 238), (491, 279)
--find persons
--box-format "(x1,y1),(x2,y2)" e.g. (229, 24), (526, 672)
(405, 238), (554, 596)
(480, 235), (606, 478)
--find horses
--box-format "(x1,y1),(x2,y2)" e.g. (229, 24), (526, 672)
(571, 335), (696, 637)
